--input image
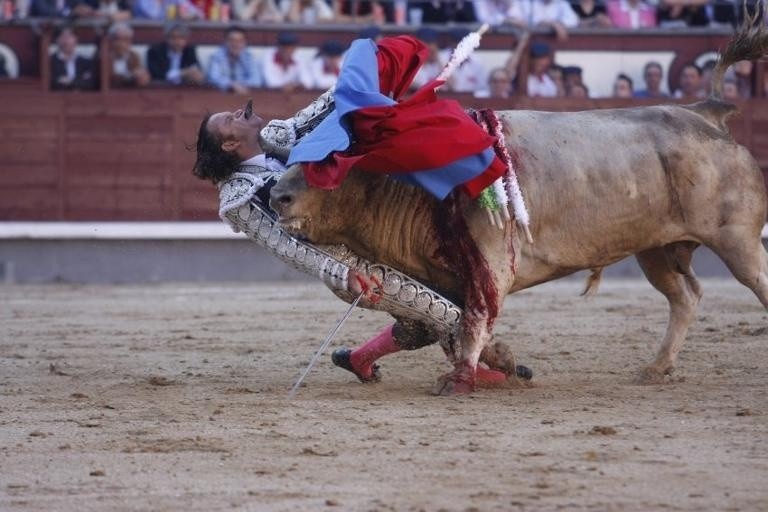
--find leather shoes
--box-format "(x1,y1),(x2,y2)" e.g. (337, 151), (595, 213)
(332, 346), (384, 383)
(512, 361), (533, 383)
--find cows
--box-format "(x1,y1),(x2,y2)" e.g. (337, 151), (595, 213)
(269, 0), (768, 395)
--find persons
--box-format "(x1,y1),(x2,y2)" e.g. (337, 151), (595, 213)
(193, 83), (533, 384)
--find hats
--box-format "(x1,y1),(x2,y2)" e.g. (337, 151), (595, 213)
(277, 21), (555, 59)
